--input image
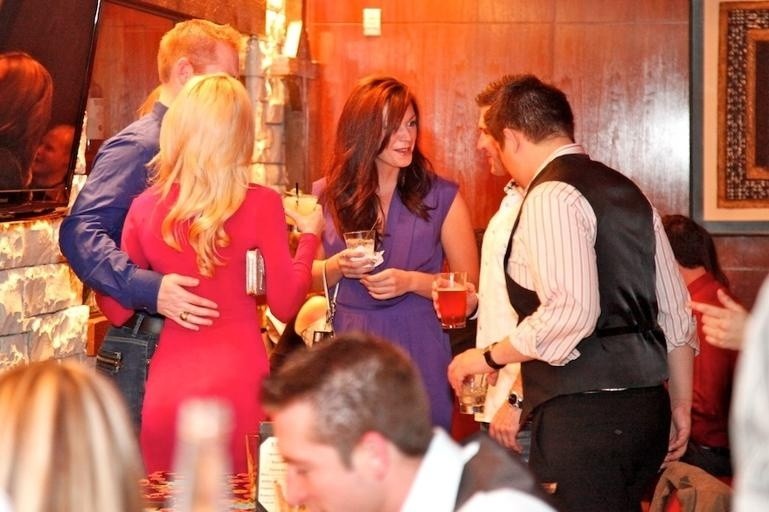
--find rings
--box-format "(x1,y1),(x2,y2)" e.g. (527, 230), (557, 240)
(179, 312), (187, 322)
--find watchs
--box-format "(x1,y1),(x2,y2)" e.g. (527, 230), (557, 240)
(506, 390), (524, 409)
(482, 343), (505, 370)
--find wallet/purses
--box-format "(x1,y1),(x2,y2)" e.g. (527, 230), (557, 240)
(246, 248), (265, 297)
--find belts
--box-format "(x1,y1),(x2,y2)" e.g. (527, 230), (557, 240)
(124, 314), (162, 333)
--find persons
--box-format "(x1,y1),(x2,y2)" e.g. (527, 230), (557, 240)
(447, 81), (699, 512)
(262, 332), (551, 512)
(57, 19), (244, 436)
(729, 276), (769, 512)
(471, 73), (525, 456)
(1, 361), (149, 511)
(28, 124), (77, 213)
(660, 216), (737, 476)
(311, 77), (479, 433)
(0, 53), (52, 219)
(688, 284), (747, 351)
(93, 71), (324, 512)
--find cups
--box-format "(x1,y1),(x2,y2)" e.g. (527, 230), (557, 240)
(342, 230), (377, 264)
(432, 272), (469, 330)
(282, 195), (318, 226)
(458, 372), (489, 416)
